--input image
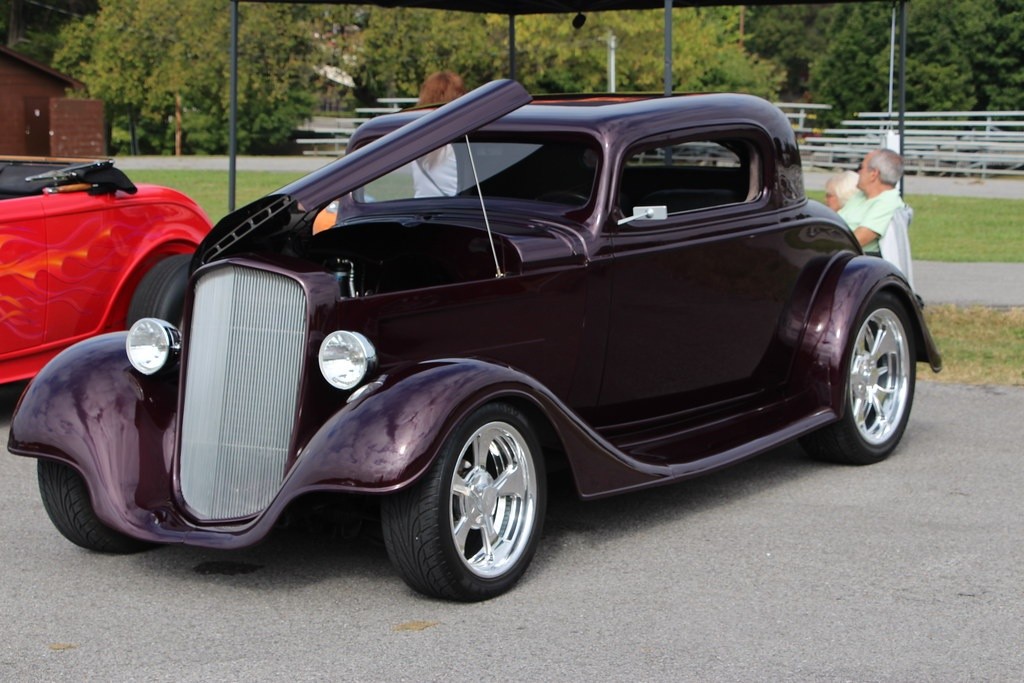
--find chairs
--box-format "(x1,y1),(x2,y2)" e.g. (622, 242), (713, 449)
(877, 207), (915, 292)
(642, 189), (745, 211)
(0, 165), (66, 195)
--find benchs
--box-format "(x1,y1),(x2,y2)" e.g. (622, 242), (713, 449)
(297, 98), (1024, 180)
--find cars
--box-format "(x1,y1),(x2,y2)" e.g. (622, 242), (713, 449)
(0, 78), (943, 604)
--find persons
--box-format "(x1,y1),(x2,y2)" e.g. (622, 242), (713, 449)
(411, 70), (466, 199)
(824, 147), (916, 295)
